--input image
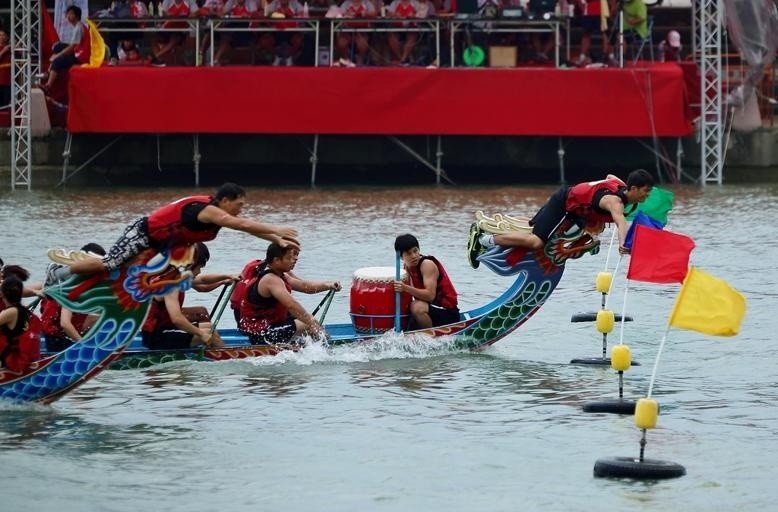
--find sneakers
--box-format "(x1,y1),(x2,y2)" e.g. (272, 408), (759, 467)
(467, 222), (485, 252)
(44, 261), (64, 287)
(467, 231), (484, 270)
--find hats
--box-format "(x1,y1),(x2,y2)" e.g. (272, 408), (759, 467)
(667, 29), (681, 49)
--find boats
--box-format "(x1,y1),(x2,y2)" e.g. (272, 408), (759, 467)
(38, 209), (602, 370)
(0, 237), (198, 408)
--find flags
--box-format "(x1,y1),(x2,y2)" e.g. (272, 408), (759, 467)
(621, 186), (675, 224)
(622, 209), (665, 256)
(626, 224), (696, 285)
(667, 266), (746, 337)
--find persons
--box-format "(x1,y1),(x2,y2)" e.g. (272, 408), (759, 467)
(230, 236), (340, 328)
(394, 232), (460, 330)
(180, 243), (235, 322)
(468, 169), (654, 269)
(42, 183), (301, 289)
(40, 243), (107, 352)
(240, 244), (342, 346)
(141, 247), (244, 350)
(0, 259), (48, 384)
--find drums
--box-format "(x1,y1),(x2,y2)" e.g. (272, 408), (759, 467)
(350, 267), (411, 334)
